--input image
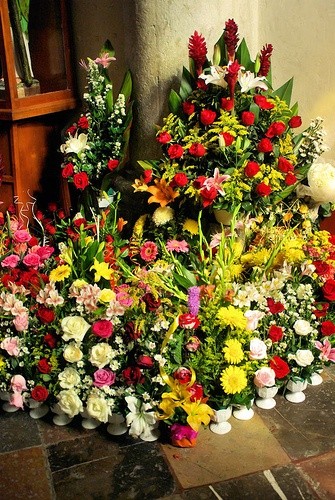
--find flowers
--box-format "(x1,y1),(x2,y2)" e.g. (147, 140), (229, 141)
(0, 16), (335, 448)
